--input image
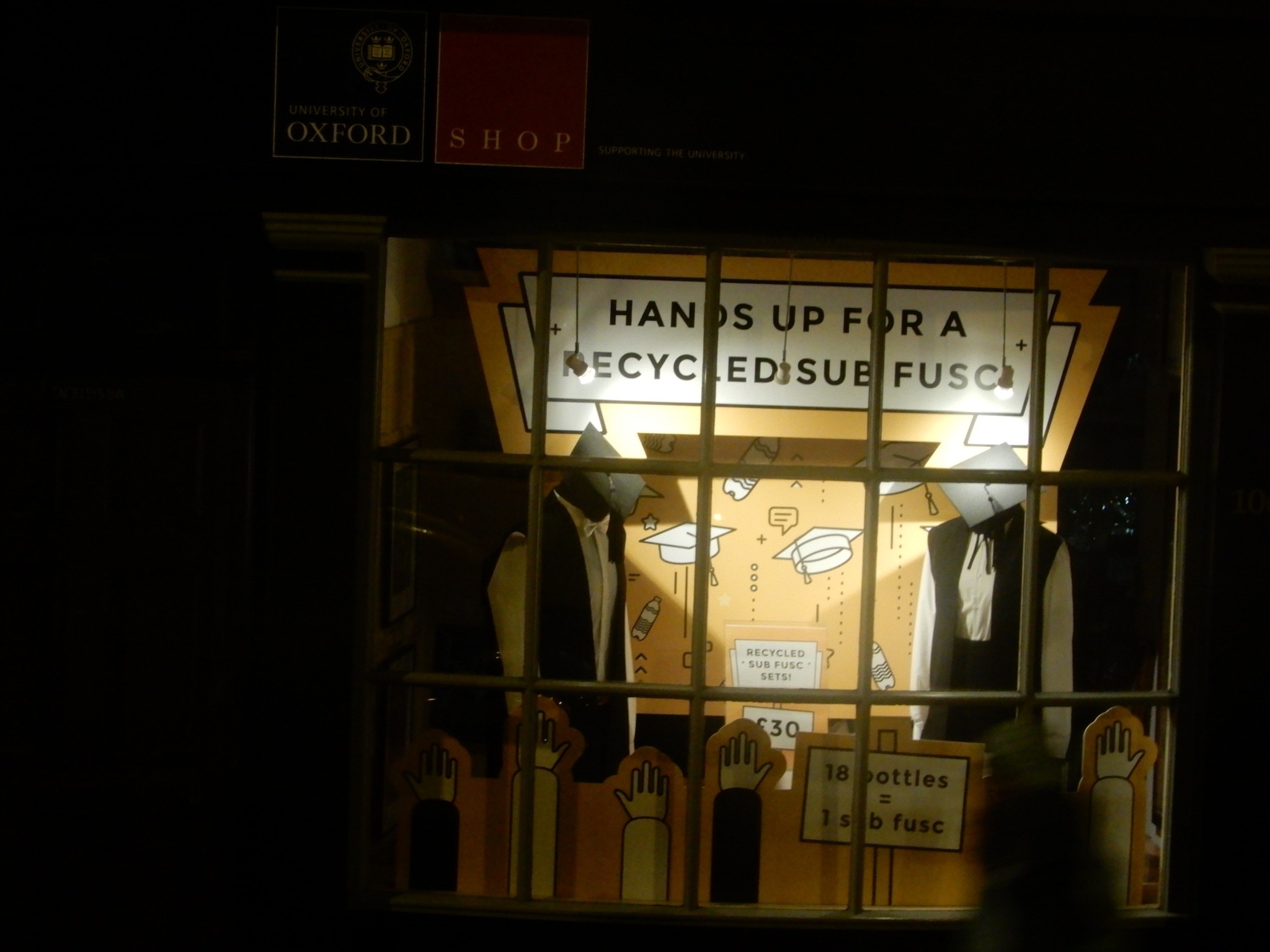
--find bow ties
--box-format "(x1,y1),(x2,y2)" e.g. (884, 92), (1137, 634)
(583, 513), (610, 537)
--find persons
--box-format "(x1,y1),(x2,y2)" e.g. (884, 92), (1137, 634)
(909, 505), (1075, 762)
(489, 474), (647, 782)
